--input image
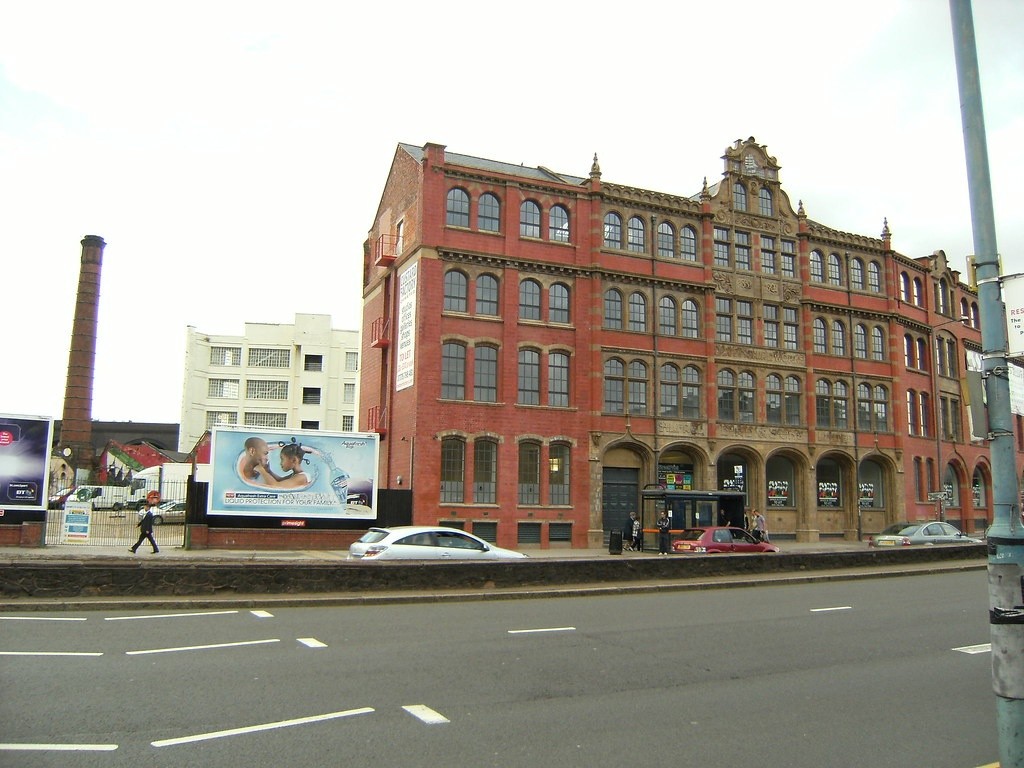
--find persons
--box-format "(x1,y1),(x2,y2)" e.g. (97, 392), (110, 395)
(719, 509), (731, 526)
(745, 507), (766, 542)
(240, 436), (310, 490)
(623, 511), (643, 551)
(128, 504), (158, 554)
(656, 510), (671, 555)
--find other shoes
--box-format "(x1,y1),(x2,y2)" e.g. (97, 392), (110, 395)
(658, 552), (663, 556)
(150, 550), (159, 554)
(663, 552), (668, 556)
(127, 548), (136, 554)
(629, 546), (634, 552)
(623, 546), (628, 552)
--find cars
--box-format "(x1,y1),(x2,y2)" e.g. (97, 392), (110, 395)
(868, 519), (983, 549)
(668, 526), (781, 553)
(347, 525), (531, 561)
(137, 500), (187, 526)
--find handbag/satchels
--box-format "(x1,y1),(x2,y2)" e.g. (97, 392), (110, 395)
(636, 532), (643, 539)
(760, 530), (770, 543)
(660, 527), (669, 533)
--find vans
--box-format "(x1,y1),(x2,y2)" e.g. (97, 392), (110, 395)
(49, 486), (78, 511)
(67, 486), (128, 511)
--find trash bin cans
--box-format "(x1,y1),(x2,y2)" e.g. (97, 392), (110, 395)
(609, 529), (623, 554)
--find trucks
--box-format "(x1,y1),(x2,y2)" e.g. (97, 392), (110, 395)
(128, 462), (211, 510)
